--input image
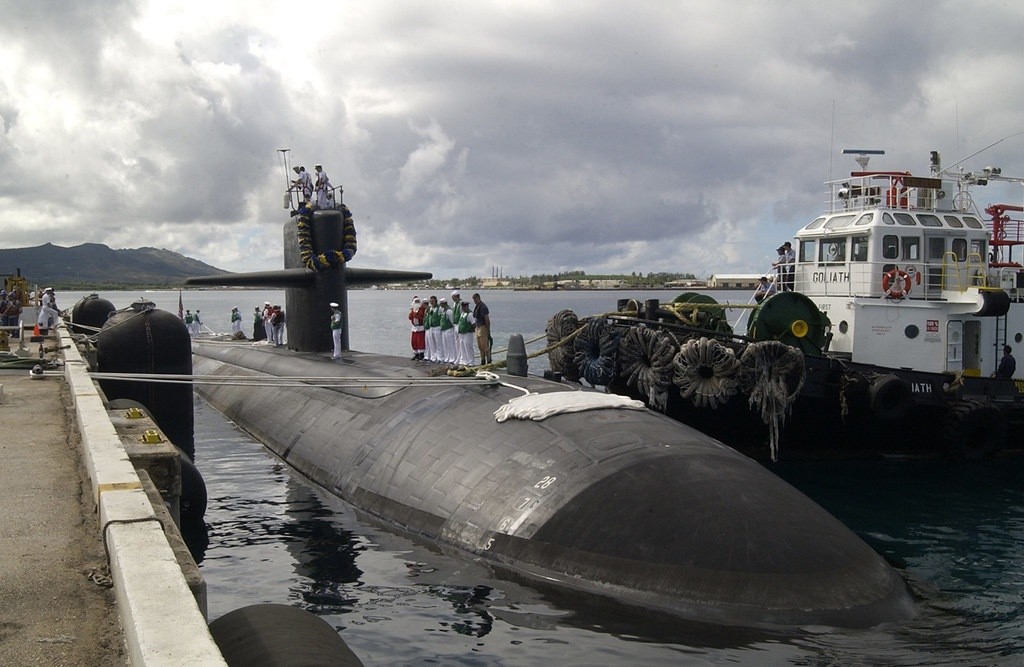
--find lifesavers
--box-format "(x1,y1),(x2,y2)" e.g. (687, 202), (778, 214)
(944, 398), (1008, 461)
(669, 336), (743, 411)
(616, 322), (677, 399)
(545, 310), (580, 373)
(867, 376), (912, 423)
(881, 269), (912, 299)
(571, 317), (622, 386)
(737, 340), (807, 413)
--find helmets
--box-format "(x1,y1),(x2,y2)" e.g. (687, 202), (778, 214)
(8, 291), (16, 297)
(0, 290), (7, 296)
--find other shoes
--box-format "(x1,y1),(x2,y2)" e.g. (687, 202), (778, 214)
(411, 353), (425, 360)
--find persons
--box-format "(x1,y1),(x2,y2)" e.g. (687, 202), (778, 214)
(25, 289), (33, 303)
(998, 345), (1016, 378)
(0, 290), (23, 338)
(231, 306), (241, 333)
(183, 310), (201, 339)
(834, 255), (845, 266)
(755, 277), (775, 305)
(473, 293), (492, 365)
(255, 302), (285, 347)
(409, 291), (476, 367)
(330, 303), (343, 359)
(291, 164), (334, 209)
(38, 287), (58, 327)
(772, 241), (796, 291)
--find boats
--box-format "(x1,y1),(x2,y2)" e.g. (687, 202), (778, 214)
(541, 145), (1024, 463)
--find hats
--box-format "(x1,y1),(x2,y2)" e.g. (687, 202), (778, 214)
(412, 295), (419, 302)
(758, 277), (767, 282)
(450, 291), (460, 297)
(421, 298), (429, 302)
(330, 302), (340, 307)
(460, 301), (471, 305)
(265, 302), (270, 306)
(44, 287), (52, 292)
(315, 164), (322, 166)
(775, 247), (785, 251)
(438, 298), (447, 303)
(783, 242), (791, 246)
